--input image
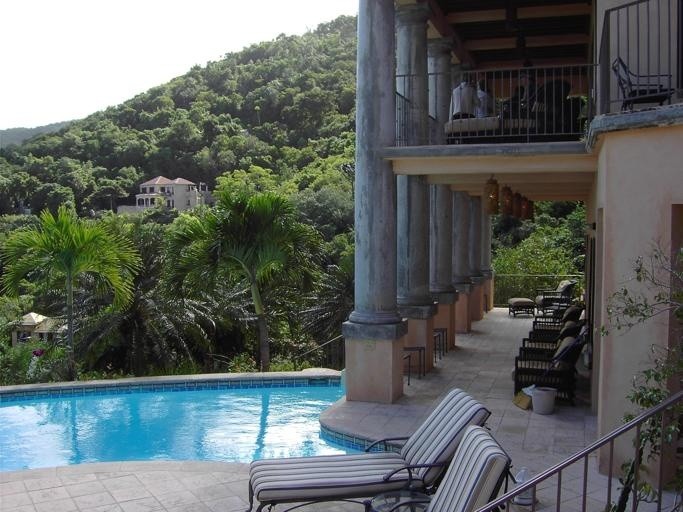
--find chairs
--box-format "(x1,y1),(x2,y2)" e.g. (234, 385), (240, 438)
(248, 389), (517, 511)
(513, 305), (587, 399)
(535, 279), (577, 316)
(611, 56), (673, 111)
(444, 79), (587, 144)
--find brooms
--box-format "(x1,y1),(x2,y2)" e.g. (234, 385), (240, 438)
(512, 331), (587, 410)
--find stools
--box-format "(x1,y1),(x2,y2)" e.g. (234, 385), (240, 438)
(508, 297), (534, 317)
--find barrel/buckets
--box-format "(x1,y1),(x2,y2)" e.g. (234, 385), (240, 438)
(513, 466), (536, 504)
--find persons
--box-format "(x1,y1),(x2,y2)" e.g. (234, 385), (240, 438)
(451, 72), (481, 144)
(471, 79), (492, 137)
(501, 85), (524, 119)
